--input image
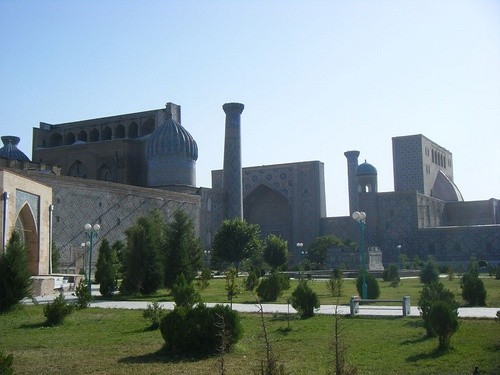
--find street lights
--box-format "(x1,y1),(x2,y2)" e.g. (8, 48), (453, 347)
(81, 241), (89, 279)
(296, 242), (304, 278)
(397, 244), (401, 279)
(352, 211), (367, 299)
(84, 223), (100, 298)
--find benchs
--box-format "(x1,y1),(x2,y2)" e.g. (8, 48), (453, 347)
(350, 295), (411, 316)
